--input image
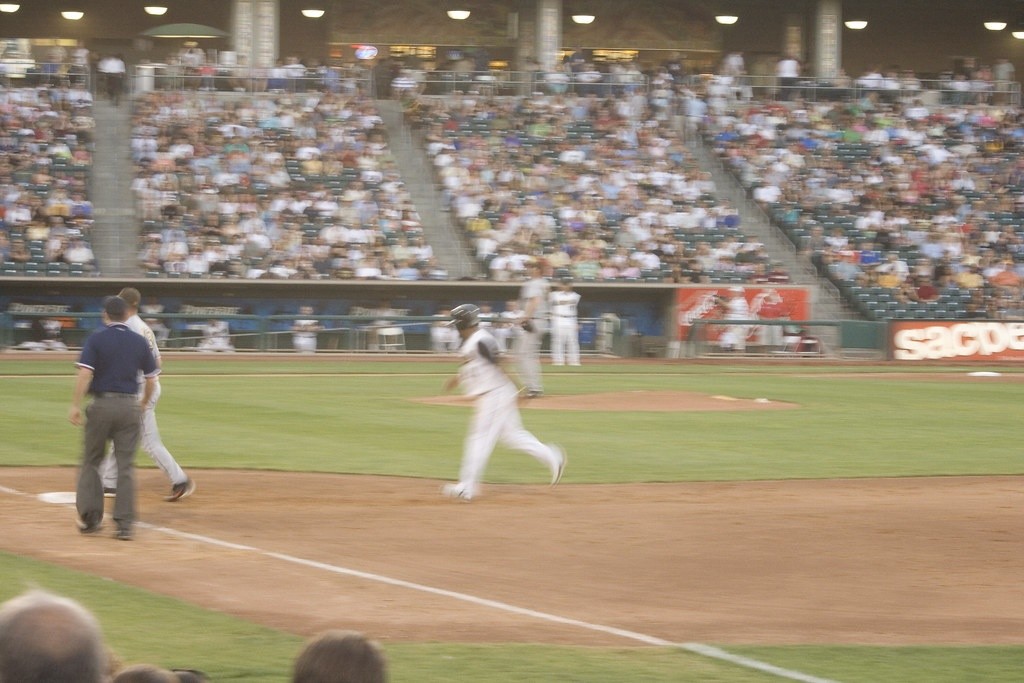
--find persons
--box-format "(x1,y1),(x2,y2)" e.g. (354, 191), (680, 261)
(292, 628), (390, 683)
(513, 259), (551, 398)
(547, 276), (583, 367)
(64, 294), (161, 541)
(0, 37), (125, 265)
(97, 286), (198, 502)
(682, 52), (1024, 319)
(141, 286), (754, 354)
(390, 52), (792, 284)
(0, 590), (213, 683)
(132, 44), (437, 280)
(433, 302), (568, 502)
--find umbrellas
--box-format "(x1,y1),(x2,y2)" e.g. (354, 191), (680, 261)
(138, 23), (231, 38)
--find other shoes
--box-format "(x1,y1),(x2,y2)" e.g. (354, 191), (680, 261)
(79, 513), (100, 533)
(550, 449), (567, 487)
(164, 478), (195, 502)
(443, 483), (481, 501)
(112, 522), (132, 540)
(103, 486), (117, 497)
(526, 390), (543, 398)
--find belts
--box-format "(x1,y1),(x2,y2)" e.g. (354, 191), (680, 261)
(95, 391), (137, 398)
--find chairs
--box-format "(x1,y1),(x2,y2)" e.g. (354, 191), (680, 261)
(422, 94), (1024, 325)
(142, 161), (357, 281)
(3, 157), (84, 278)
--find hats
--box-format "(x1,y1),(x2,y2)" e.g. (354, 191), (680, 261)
(115, 287), (141, 310)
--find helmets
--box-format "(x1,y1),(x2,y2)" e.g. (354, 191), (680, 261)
(445, 304), (480, 328)
